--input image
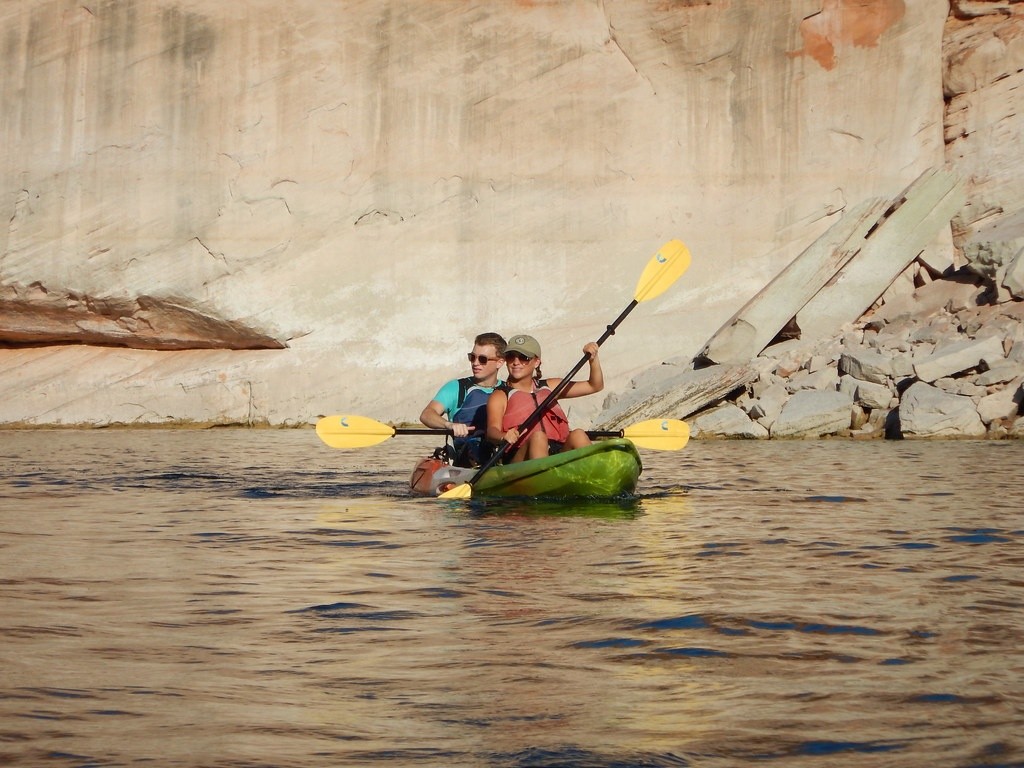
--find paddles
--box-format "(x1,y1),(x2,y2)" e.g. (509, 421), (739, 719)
(316, 415), (692, 452)
(437, 238), (693, 499)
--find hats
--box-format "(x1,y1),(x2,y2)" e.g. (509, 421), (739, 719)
(503, 335), (541, 359)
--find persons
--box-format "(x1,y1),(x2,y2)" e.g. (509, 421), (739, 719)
(486, 335), (604, 464)
(420, 332), (508, 467)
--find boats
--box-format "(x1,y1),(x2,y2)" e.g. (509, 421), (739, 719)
(411, 438), (643, 504)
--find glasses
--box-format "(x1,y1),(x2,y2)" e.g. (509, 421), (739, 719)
(506, 354), (532, 365)
(467, 353), (498, 364)
